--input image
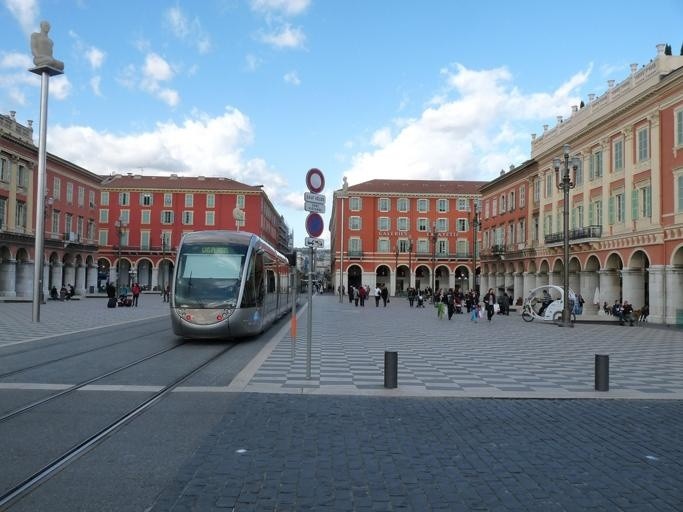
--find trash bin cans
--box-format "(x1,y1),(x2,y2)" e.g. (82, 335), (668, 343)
(90, 286), (94, 293)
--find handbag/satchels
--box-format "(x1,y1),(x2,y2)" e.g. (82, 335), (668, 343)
(494, 303), (501, 313)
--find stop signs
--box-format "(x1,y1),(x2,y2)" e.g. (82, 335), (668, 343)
(429, 227), (438, 303)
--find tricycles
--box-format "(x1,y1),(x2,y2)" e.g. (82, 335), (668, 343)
(522, 285), (576, 324)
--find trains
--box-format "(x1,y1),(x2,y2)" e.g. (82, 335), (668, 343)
(169, 229), (302, 341)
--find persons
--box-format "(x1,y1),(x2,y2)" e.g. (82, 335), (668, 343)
(573, 294), (585, 315)
(537, 290), (554, 316)
(407, 286), (513, 323)
(51, 284), (74, 301)
(338, 285), (345, 299)
(603, 300), (649, 326)
(163, 280), (170, 303)
(313, 283), (324, 294)
(348, 282), (390, 307)
(106, 281), (141, 307)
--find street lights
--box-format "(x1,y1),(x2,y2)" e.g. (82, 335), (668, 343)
(160, 232), (168, 294)
(468, 203), (482, 291)
(407, 235), (413, 287)
(40, 190), (54, 304)
(114, 216), (127, 297)
(553, 143), (580, 327)
(392, 243), (401, 296)
